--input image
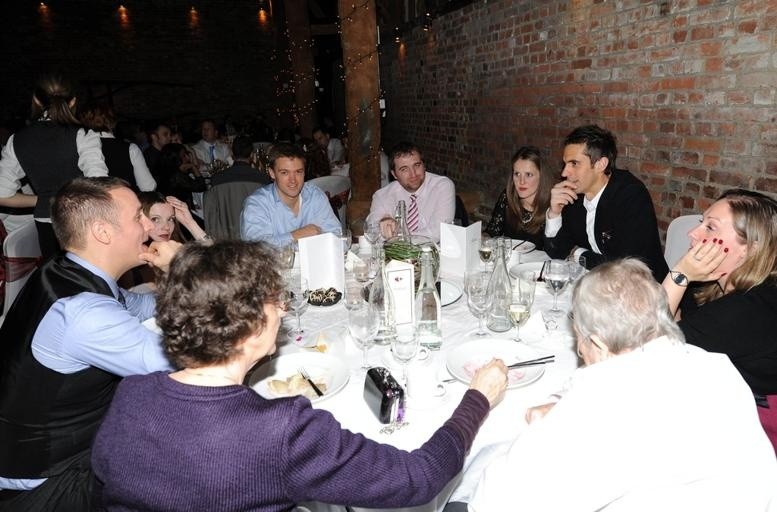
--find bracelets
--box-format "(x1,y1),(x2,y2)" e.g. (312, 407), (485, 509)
(667, 271), (692, 286)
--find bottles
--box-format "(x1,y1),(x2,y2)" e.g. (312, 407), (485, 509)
(256, 144), (270, 175)
(393, 200), (411, 244)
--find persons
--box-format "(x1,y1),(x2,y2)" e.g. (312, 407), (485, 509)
(239, 143), (344, 252)
(140, 120), (267, 213)
(363, 140), (456, 246)
(542, 124), (672, 282)
(0, 70), (160, 260)
(296, 127), (349, 211)
(467, 248), (777, 512)
(661, 188), (777, 455)
(133, 191), (215, 276)
(0, 176), (184, 511)
(92, 238), (510, 512)
(481, 144), (551, 244)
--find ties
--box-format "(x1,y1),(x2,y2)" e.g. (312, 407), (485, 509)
(407, 194), (420, 234)
(209, 146), (215, 165)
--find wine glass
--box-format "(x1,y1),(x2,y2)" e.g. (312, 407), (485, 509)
(544, 259), (571, 318)
(272, 239), (536, 360)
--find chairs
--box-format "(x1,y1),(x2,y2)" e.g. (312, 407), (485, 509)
(1, 221), (41, 314)
(309, 175), (352, 232)
(200, 181), (262, 240)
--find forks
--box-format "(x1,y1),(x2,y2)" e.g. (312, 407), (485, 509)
(298, 366), (325, 398)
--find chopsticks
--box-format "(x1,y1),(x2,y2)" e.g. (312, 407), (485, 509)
(442, 355), (556, 384)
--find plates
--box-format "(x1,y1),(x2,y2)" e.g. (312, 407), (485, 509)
(510, 261), (578, 286)
(484, 239), (536, 252)
(248, 351), (351, 401)
(445, 339), (546, 389)
